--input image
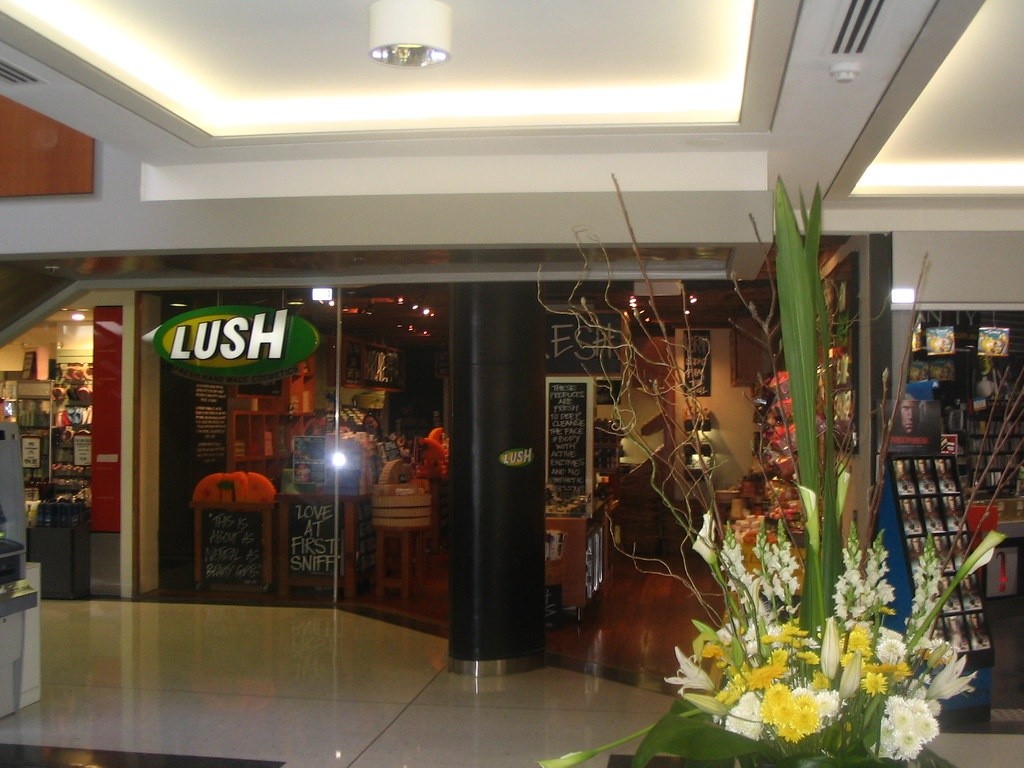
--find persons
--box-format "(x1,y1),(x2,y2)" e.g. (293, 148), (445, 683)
(901, 401), (919, 434)
(363, 415), (391, 443)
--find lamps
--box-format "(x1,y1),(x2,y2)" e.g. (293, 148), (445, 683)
(292, 461), (325, 485)
(367, 0), (451, 70)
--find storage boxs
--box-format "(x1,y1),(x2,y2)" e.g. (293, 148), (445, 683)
(292, 435), (326, 462)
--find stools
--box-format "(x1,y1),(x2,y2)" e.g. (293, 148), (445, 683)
(374, 526), (433, 598)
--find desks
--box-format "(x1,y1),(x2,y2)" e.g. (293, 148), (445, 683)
(273, 492), (373, 599)
(187, 501), (274, 587)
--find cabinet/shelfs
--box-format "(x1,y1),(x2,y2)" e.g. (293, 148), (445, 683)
(226, 360), (315, 478)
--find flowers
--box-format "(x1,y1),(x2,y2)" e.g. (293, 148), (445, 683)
(539, 172), (1001, 768)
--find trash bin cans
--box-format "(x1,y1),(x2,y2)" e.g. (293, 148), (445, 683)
(0, 536), (39, 718)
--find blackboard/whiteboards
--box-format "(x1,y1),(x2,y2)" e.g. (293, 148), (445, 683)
(546, 381), (594, 513)
(191, 500), (275, 592)
(278, 494), (353, 590)
